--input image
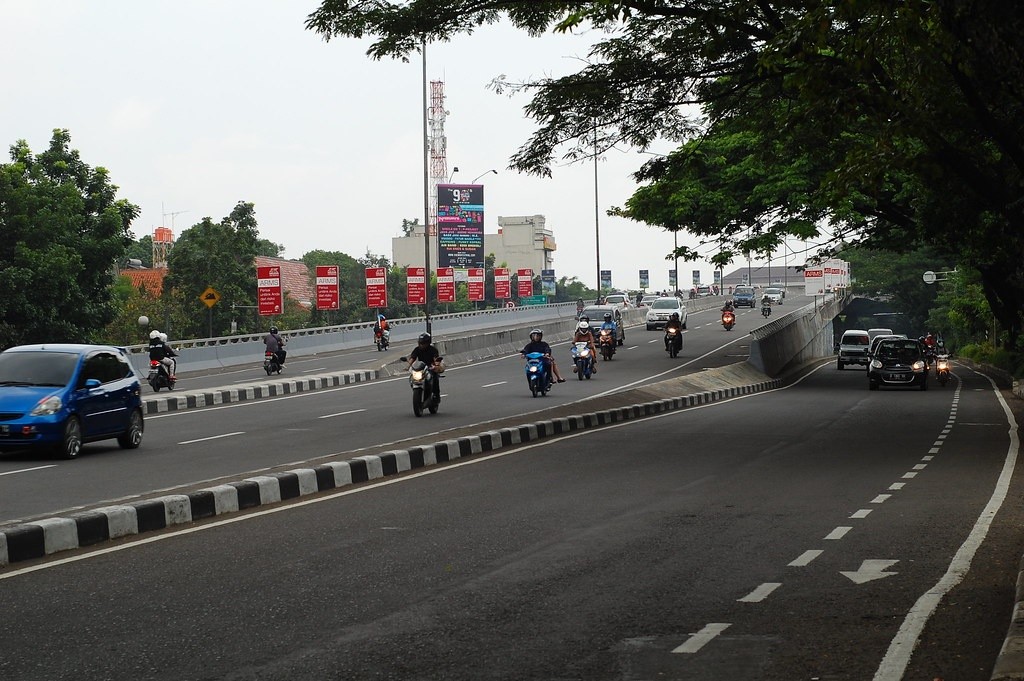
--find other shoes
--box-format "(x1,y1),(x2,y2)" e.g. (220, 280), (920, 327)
(280, 364), (287, 369)
(557, 378), (566, 383)
(550, 380), (556, 384)
(573, 367), (578, 373)
(592, 366), (597, 374)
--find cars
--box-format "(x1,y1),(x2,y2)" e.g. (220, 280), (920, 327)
(864, 329), (907, 376)
(604, 295), (630, 308)
(574, 305), (626, 346)
(761, 288), (783, 305)
(697, 286), (712, 298)
(735, 284), (762, 289)
(867, 339), (932, 391)
(640, 296), (660, 305)
(0, 342), (146, 458)
(732, 286), (756, 308)
(770, 283), (785, 298)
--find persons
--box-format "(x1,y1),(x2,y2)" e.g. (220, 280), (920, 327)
(729, 286), (733, 294)
(661, 289), (695, 298)
(572, 315), (598, 374)
(263, 327), (287, 369)
(636, 291), (643, 302)
(664, 312), (683, 351)
(149, 330), (179, 380)
(761, 295), (772, 315)
(374, 315), (390, 343)
(403, 333), (441, 403)
(599, 313), (617, 354)
(599, 294), (605, 305)
(576, 297), (585, 312)
(521, 329), (566, 384)
(721, 300), (736, 325)
(712, 285), (719, 296)
(919, 334), (951, 380)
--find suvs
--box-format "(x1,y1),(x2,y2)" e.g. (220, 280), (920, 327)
(646, 297), (688, 330)
(836, 330), (873, 370)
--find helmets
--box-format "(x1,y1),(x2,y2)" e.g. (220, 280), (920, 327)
(579, 321), (589, 335)
(579, 315), (589, 324)
(919, 336), (925, 342)
(927, 334), (932, 338)
(937, 339), (945, 347)
(150, 330), (168, 343)
(431, 357), (446, 373)
(530, 329), (543, 341)
(725, 300), (732, 305)
(377, 315), (385, 320)
(669, 312), (679, 321)
(418, 332), (431, 344)
(270, 326), (278, 334)
(604, 313), (611, 322)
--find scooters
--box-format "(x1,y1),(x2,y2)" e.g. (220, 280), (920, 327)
(577, 308), (582, 316)
(926, 345), (933, 363)
(598, 329), (615, 361)
(147, 347), (181, 392)
(721, 308), (734, 332)
(519, 350), (551, 398)
(263, 338), (288, 376)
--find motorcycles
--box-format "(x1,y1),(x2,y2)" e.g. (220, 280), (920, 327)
(663, 327), (681, 359)
(762, 303), (771, 318)
(571, 341), (594, 381)
(400, 357), (446, 417)
(934, 354), (952, 385)
(374, 323), (393, 351)
